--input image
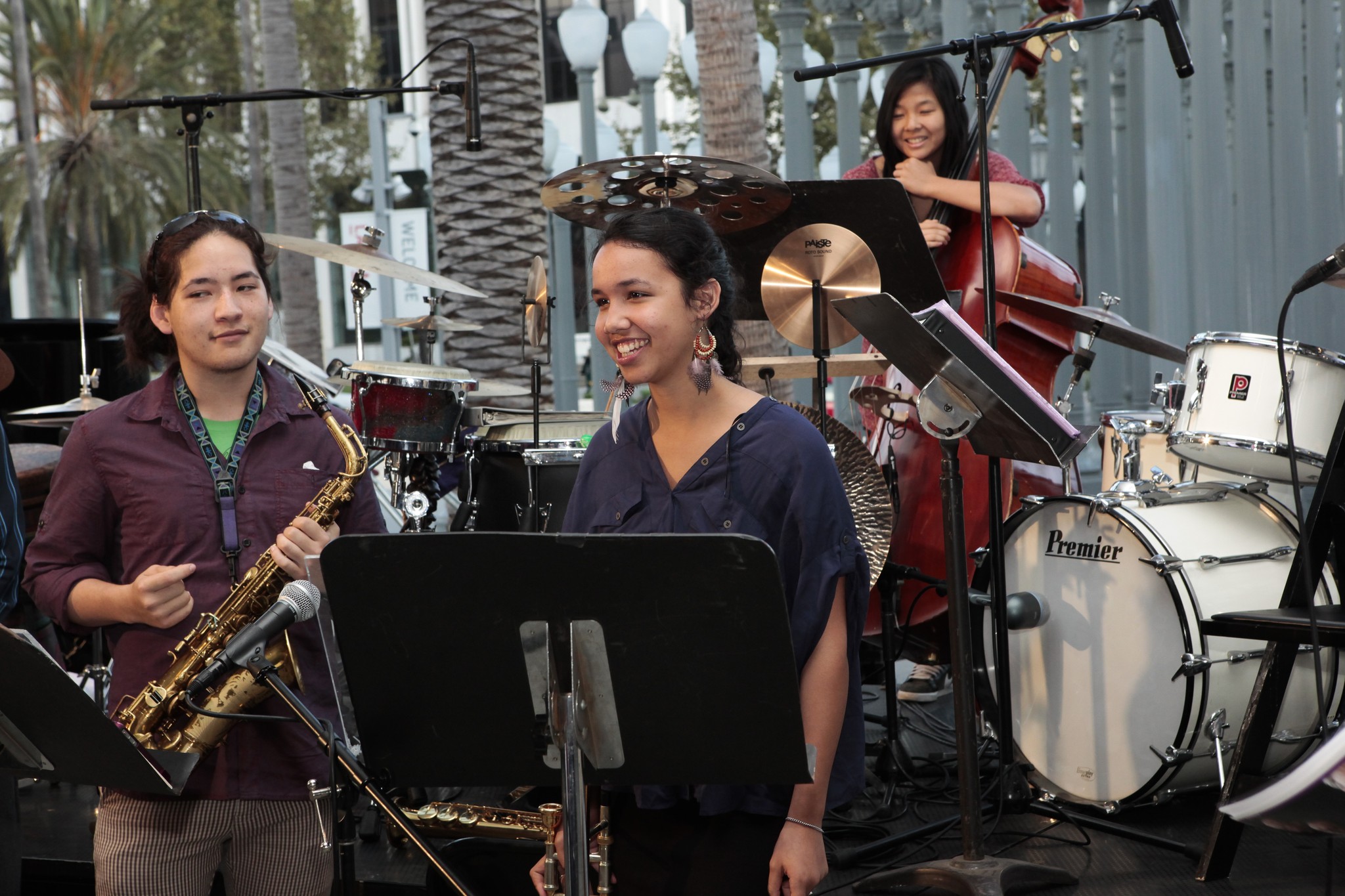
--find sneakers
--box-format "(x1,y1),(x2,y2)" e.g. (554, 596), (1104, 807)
(896, 653), (953, 701)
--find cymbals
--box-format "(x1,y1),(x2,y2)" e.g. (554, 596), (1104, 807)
(776, 398), (893, 594)
(759, 220), (884, 349)
(540, 154), (792, 239)
(3, 398), (110, 429)
(258, 229), (489, 300)
(521, 254), (549, 347)
(379, 315), (485, 333)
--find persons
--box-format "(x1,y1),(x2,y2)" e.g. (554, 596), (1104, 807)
(18, 210), (392, 896)
(531, 207), (870, 895)
(839, 58), (1046, 702)
(0, 345), (67, 675)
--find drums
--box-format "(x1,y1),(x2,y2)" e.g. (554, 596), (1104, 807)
(1167, 330), (1345, 486)
(345, 356), (479, 456)
(1099, 409), (1261, 494)
(6, 441), (66, 539)
(458, 408), (617, 534)
(964, 471), (1344, 814)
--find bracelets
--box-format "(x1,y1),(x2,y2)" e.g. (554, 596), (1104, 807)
(784, 816), (825, 834)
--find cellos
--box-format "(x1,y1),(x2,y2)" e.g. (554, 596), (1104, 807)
(851, 0), (1090, 660)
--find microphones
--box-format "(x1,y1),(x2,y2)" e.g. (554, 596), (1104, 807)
(1292, 243), (1345, 294)
(1150, 0), (1194, 78)
(463, 44), (483, 153)
(185, 579), (321, 696)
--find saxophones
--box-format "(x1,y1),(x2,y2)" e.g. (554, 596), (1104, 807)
(110, 374), (369, 765)
(358, 776), (602, 848)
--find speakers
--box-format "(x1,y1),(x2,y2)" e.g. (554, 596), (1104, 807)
(1, 318), (152, 411)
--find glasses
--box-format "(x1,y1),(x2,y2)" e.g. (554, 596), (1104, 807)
(152, 210), (251, 286)
